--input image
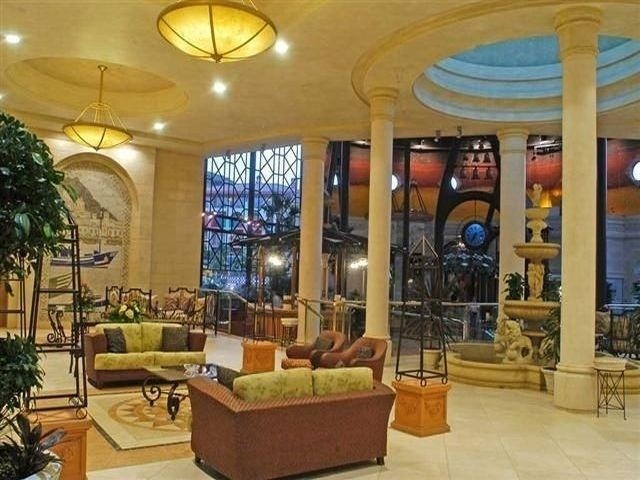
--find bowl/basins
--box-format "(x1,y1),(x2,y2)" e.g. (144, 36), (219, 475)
(184, 363), (199, 370)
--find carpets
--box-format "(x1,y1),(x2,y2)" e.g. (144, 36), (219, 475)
(26, 385), (197, 470)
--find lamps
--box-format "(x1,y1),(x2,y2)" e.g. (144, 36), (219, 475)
(59, 65), (134, 152)
(155, 0), (277, 65)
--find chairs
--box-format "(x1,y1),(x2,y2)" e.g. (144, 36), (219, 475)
(280, 329), (388, 382)
(104, 285), (219, 336)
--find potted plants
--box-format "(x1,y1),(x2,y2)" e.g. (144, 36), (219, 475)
(399, 273), (465, 371)
(533, 281), (562, 396)
(0, 111), (81, 480)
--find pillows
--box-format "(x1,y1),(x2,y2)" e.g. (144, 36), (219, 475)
(217, 366), (244, 393)
(161, 325), (190, 351)
(102, 327), (126, 353)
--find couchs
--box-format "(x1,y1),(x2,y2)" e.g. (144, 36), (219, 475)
(186, 365), (395, 480)
(82, 322), (208, 390)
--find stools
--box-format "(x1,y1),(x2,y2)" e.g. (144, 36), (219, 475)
(592, 356), (628, 420)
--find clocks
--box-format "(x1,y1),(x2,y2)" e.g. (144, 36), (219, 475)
(461, 222), (489, 250)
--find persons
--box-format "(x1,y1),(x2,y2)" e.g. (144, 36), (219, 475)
(531, 183), (543, 206)
(526, 263), (546, 298)
